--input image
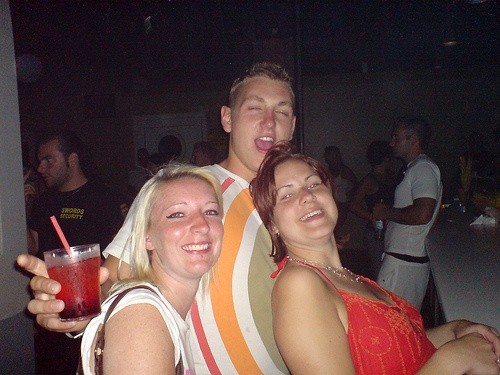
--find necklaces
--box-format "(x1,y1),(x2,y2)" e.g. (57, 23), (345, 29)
(285, 254), (365, 285)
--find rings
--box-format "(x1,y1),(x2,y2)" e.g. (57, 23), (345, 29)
(496, 359), (500, 362)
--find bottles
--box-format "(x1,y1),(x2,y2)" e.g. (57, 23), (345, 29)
(373, 199), (385, 241)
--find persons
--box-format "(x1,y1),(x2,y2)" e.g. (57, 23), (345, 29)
(77, 162), (225, 375)
(249, 139), (500, 375)
(15, 61), (298, 375)
(19, 113), (500, 375)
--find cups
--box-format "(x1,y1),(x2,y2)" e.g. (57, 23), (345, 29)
(44, 244), (102, 322)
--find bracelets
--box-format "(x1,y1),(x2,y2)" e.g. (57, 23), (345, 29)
(66, 331), (86, 340)
